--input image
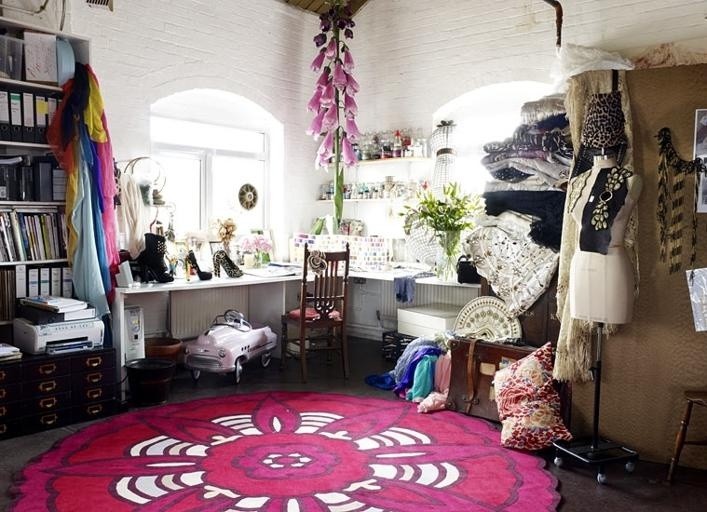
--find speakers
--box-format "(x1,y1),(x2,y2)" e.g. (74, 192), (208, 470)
(117, 260), (134, 286)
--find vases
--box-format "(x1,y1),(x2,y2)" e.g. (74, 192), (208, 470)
(435, 231), (463, 282)
(252, 249), (262, 268)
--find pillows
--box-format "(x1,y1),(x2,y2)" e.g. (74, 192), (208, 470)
(492, 342), (573, 449)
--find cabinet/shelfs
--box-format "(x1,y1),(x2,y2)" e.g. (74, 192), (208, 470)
(0, 16), (93, 327)
(317, 155), (430, 203)
(0, 343), (118, 440)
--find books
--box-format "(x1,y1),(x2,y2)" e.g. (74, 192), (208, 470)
(0, 151), (97, 361)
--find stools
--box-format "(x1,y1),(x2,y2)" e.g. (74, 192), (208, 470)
(667, 389), (707, 482)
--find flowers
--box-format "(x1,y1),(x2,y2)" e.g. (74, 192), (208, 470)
(398, 182), (485, 256)
(241, 236), (272, 262)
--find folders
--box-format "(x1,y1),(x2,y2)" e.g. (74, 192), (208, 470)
(0, 87), (63, 144)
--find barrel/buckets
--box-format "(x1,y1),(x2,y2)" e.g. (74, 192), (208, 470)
(125, 358), (175, 404)
(145, 337), (184, 359)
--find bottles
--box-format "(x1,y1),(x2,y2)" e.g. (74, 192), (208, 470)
(349, 128), (429, 160)
(319, 176), (428, 202)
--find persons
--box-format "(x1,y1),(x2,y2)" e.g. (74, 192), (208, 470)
(565, 152), (645, 326)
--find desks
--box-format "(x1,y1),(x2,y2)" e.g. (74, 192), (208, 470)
(113, 268), (319, 402)
(321, 264), (483, 346)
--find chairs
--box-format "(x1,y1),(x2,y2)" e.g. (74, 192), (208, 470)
(280, 240), (351, 383)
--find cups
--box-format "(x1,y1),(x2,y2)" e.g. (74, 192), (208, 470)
(392, 238), (407, 263)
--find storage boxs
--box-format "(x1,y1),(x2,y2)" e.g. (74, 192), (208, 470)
(398, 302), (464, 342)
(481, 263), (561, 348)
(446, 338), (559, 423)
(382, 331), (419, 372)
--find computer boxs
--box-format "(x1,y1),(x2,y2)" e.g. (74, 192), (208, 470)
(125, 305), (146, 390)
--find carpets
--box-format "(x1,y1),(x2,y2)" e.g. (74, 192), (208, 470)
(6, 392), (563, 512)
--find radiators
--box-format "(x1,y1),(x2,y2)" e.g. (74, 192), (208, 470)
(169, 286), (250, 340)
(380, 280), (479, 334)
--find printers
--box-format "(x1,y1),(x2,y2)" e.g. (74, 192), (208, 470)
(14, 317), (104, 354)
